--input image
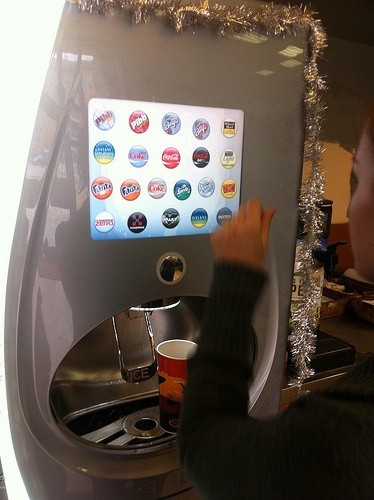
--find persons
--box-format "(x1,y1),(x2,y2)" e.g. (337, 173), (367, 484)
(174, 105), (374, 499)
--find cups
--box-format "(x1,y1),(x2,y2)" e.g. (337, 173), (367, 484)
(154, 339), (198, 435)
